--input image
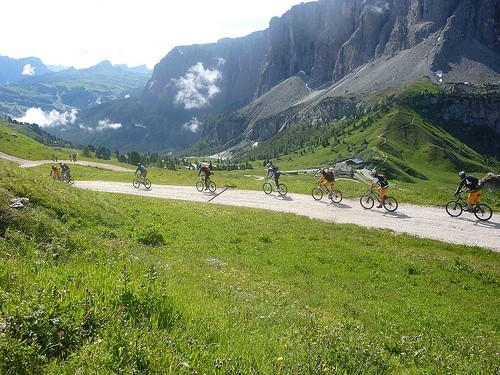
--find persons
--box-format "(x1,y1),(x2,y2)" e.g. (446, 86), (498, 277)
(197, 164), (210, 189)
(266, 163), (280, 191)
(51, 163), (71, 180)
(316, 168), (335, 196)
(455, 171), (480, 210)
(372, 172), (389, 208)
(134, 163), (147, 183)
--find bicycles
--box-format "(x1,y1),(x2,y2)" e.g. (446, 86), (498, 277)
(196, 175), (216, 193)
(133, 174), (151, 189)
(50, 170), (74, 185)
(446, 189), (493, 221)
(312, 181), (342, 203)
(70, 157), (77, 162)
(263, 176), (288, 196)
(360, 186), (398, 212)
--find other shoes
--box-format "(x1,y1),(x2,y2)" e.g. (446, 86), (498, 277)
(376, 205), (382, 208)
(463, 208), (471, 212)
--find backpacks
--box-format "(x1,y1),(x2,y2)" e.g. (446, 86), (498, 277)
(203, 166), (210, 173)
(325, 172), (334, 180)
(271, 165), (279, 171)
(467, 176), (478, 185)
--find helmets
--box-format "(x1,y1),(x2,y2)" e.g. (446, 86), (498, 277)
(198, 164), (201, 168)
(458, 171), (465, 180)
(318, 168), (324, 173)
(267, 164), (271, 167)
(369, 169), (377, 174)
